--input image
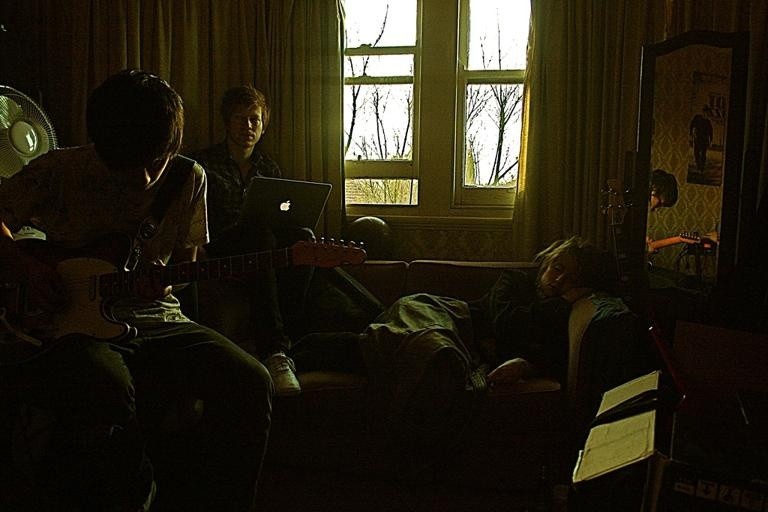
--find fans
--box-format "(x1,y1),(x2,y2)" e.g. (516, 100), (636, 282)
(1, 83), (62, 183)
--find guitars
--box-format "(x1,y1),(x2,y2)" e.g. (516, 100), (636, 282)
(600, 180), (650, 320)
(0, 237), (367, 342)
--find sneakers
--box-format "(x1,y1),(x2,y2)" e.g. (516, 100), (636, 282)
(261, 350), (305, 396)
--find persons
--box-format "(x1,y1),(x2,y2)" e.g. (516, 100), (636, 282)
(1, 67), (275, 510)
(362, 235), (602, 494)
(646, 169), (678, 253)
(189, 83), (319, 398)
(688, 104), (714, 176)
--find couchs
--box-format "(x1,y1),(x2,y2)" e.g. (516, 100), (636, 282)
(205, 256), (635, 483)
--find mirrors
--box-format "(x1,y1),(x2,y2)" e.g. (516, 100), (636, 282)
(626, 28), (753, 322)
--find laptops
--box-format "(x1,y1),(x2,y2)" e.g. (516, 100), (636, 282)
(212, 176), (332, 240)
